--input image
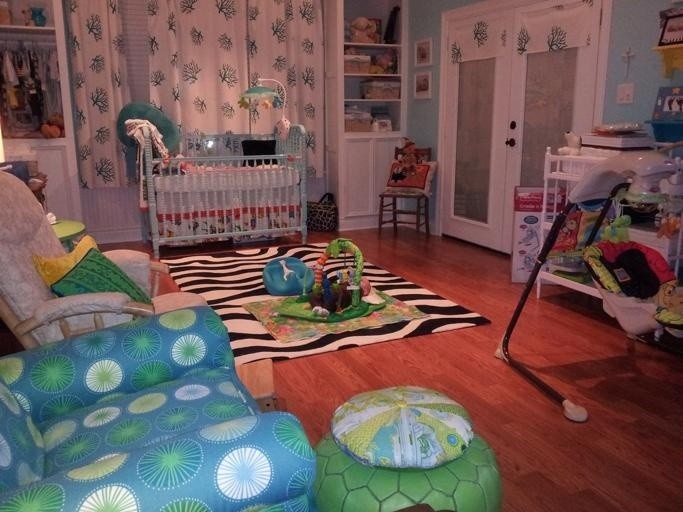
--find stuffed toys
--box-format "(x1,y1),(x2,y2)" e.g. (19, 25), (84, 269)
(237, 77), (292, 141)
(269, 238), (386, 323)
(349, 16), (377, 43)
(398, 137), (418, 176)
(557, 130), (582, 157)
(599, 192), (667, 244)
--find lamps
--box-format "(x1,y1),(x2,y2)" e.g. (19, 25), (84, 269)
(240, 77), (293, 141)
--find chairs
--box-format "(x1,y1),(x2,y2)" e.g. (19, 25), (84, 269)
(0, 170), (209, 353)
(0, 299), (325, 512)
(376, 143), (435, 241)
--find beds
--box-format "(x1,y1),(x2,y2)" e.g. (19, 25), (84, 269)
(131, 123), (312, 267)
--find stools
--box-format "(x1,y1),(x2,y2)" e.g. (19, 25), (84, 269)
(308, 384), (508, 512)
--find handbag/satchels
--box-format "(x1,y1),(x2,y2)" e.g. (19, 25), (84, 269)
(305, 192), (340, 233)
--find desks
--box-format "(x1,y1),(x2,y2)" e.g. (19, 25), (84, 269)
(45, 215), (88, 253)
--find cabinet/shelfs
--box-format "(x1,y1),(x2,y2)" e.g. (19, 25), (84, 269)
(534, 143), (681, 345)
(641, 41), (683, 142)
(0, 1), (85, 222)
(321, 0), (415, 234)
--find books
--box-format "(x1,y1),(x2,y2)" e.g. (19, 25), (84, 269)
(579, 133), (654, 149)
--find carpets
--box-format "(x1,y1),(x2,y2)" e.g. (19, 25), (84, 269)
(157, 236), (495, 372)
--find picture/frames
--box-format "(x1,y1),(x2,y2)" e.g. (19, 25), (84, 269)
(413, 36), (433, 67)
(413, 69), (432, 101)
(657, 13), (683, 46)
(651, 86), (683, 121)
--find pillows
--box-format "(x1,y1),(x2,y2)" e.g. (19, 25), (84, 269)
(382, 156), (438, 198)
(48, 246), (154, 311)
(32, 233), (100, 294)
